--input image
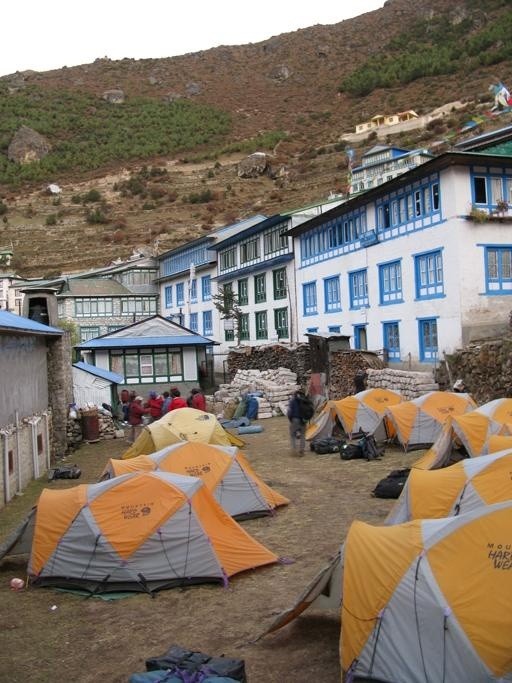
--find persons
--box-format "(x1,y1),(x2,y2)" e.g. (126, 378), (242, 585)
(113, 389), (206, 446)
(355, 369), (368, 393)
(285, 390), (309, 457)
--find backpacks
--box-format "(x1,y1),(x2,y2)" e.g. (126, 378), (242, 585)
(297, 397), (314, 419)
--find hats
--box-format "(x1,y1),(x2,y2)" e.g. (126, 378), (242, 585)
(135, 396), (143, 401)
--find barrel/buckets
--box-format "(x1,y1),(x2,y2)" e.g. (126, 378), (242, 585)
(81, 415), (99, 440)
(311, 372), (321, 395)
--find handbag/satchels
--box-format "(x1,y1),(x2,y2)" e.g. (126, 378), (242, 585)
(128, 643), (248, 683)
(374, 469), (409, 498)
(310, 429), (381, 461)
(48, 464), (81, 480)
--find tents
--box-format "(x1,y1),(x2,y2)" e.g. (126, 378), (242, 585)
(305, 389), (512, 527)
(252, 501), (512, 682)
(1, 407), (291, 599)
